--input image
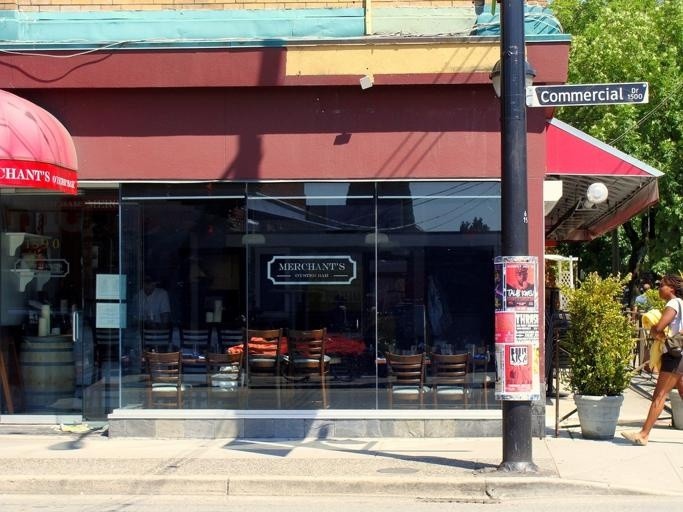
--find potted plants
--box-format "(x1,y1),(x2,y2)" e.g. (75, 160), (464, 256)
(554, 270), (641, 440)
(636, 289), (683, 431)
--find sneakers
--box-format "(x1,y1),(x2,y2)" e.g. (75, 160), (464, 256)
(621, 430), (648, 446)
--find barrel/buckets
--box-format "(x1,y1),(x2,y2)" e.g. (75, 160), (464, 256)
(19, 336), (76, 412)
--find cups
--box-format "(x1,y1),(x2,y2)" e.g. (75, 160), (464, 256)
(38, 305), (61, 336)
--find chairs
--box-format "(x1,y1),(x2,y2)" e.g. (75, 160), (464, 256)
(87, 322), (502, 420)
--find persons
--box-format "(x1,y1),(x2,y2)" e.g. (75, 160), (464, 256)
(631, 283), (650, 326)
(129, 275), (171, 351)
(621, 276), (683, 446)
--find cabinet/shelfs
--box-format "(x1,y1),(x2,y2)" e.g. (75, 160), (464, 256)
(0, 231), (54, 293)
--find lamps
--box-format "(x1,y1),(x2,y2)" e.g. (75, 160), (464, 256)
(365, 232), (389, 245)
(585, 182), (609, 209)
(241, 194), (266, 244)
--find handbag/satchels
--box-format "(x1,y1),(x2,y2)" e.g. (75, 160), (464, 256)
(664, 332), (683, 359)
